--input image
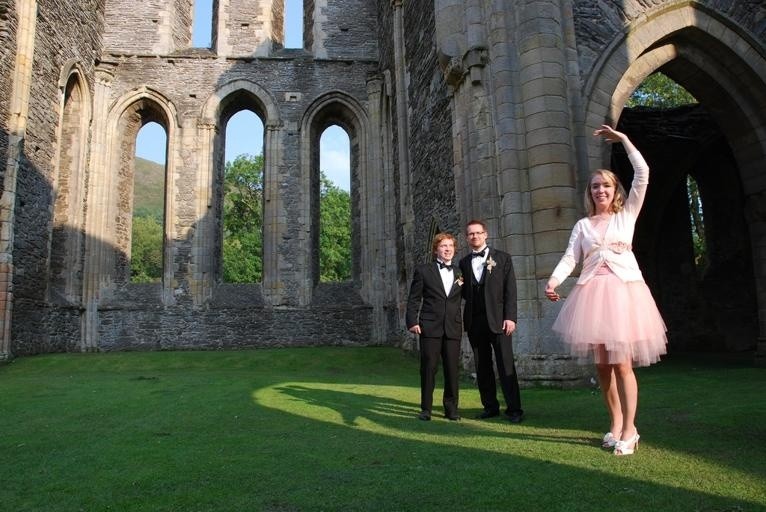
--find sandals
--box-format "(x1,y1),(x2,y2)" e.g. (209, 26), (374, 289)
(601, 433), (618, 448)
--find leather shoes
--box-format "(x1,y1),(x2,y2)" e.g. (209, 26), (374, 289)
(419, 410), (432, 420)
(508, 414), (523, 423)
(475, 411), (500, 419)
(446, 411), (460, 420)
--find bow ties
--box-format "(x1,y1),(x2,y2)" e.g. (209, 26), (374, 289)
(437, 260), (452, 271)
(471, 246), (489, 257)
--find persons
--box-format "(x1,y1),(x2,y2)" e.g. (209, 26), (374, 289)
(543, 123), (669, 458)
(405, 232), (465, 421)
(457, 220), (526, 424)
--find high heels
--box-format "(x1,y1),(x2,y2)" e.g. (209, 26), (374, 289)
(614, 428), (640, 456)
